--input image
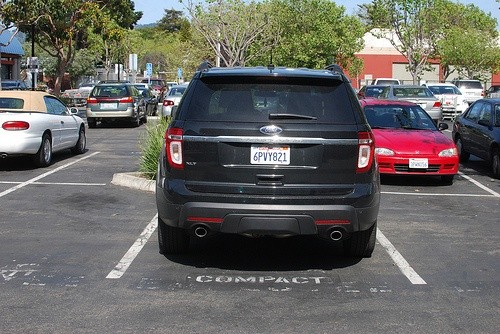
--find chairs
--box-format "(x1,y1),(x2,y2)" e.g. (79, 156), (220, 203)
(215, 89), (267, 122)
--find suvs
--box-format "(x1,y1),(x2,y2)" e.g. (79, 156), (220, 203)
(84, 80), (148, 127)
(0, 79), (27, 91)
(157, 63), (381, 257)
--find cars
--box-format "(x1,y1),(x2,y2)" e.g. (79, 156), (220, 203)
(381, 85), (443, 126)
(357, 85), (385, 101)
(452, 79), (484, 105)
(80, 82), (96, 91)
(451, 98), (500, 180)
(485, 85), (500, 98)
(360, 97), (459, 187)
(141, 77), (191, 121)
(371, 78), (400, 86)
(419, 83), (470, 119)
(0, 90), (86, 169)
